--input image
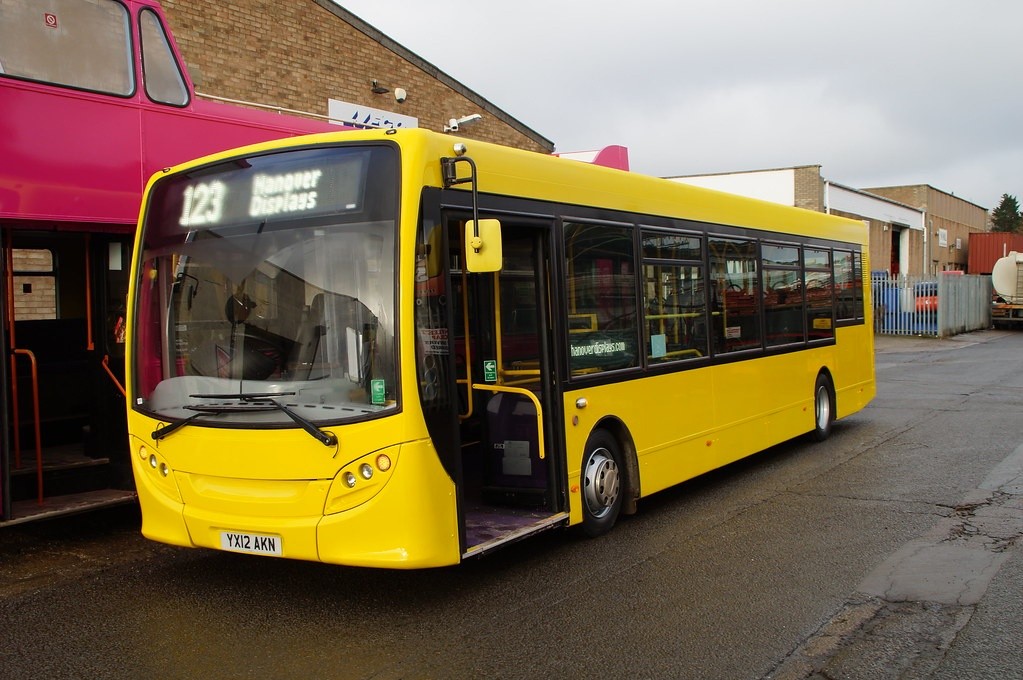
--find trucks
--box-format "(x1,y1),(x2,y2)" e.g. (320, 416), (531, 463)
(988, 249), (1023, 329)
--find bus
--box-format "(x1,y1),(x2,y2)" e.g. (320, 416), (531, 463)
(116, 126), (878, 570)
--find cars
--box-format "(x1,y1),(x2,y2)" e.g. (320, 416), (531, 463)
(912, 281), (937, 313)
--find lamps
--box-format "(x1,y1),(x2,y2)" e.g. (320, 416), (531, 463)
(372, 79), (390, 94)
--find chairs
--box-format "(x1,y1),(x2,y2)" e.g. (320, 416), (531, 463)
(717, 278), (845, 344)
